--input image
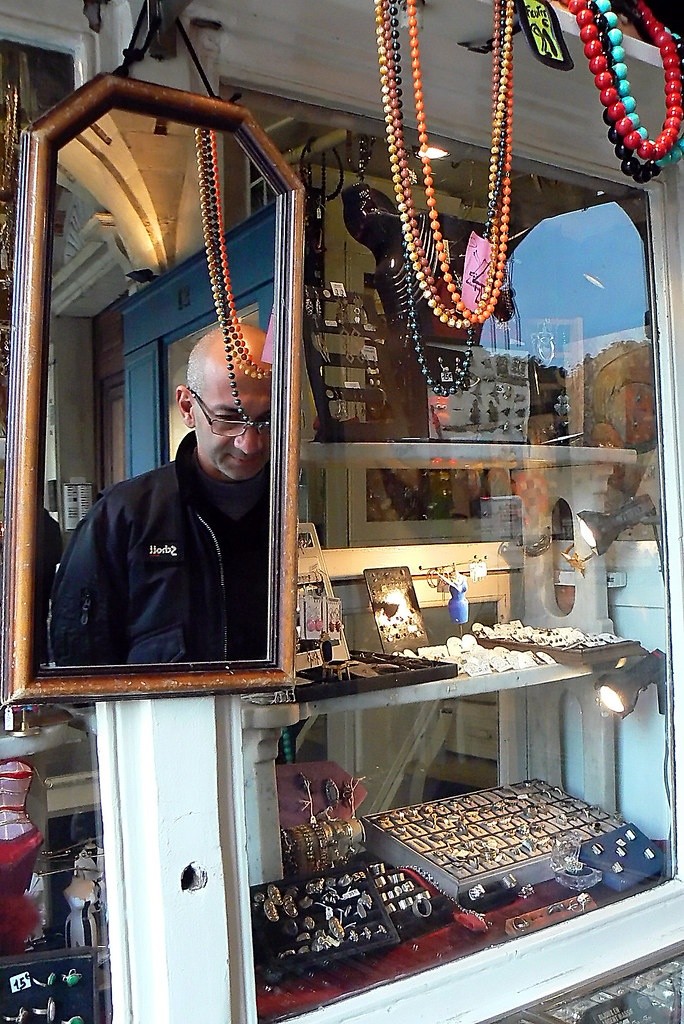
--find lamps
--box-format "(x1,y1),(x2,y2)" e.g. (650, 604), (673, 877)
(591, 649), (667, 721)
(576, 494), (660, 557)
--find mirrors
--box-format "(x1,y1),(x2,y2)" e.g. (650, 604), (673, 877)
(0, 73), (307, 708)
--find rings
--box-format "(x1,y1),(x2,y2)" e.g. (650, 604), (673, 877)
(4, 968), (85, 1024)
(257, 862), (432, 957)
(376, 780), (656, 932)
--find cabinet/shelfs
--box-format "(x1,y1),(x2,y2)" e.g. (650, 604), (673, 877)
(95, 442), (681, 1024)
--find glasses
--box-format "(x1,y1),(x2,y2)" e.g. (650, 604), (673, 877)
(186, 387), (271, 436)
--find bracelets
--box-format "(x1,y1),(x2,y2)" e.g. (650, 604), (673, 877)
(281, 820), (370, 870)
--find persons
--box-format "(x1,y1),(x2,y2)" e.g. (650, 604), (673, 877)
(51, 325), (272, 672)
(447, 571), (469, 624)
(0, 754), (105, 949)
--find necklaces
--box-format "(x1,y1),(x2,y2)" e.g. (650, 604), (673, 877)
(377, 0), (684, 395)
(195, 126), (276, 433)
